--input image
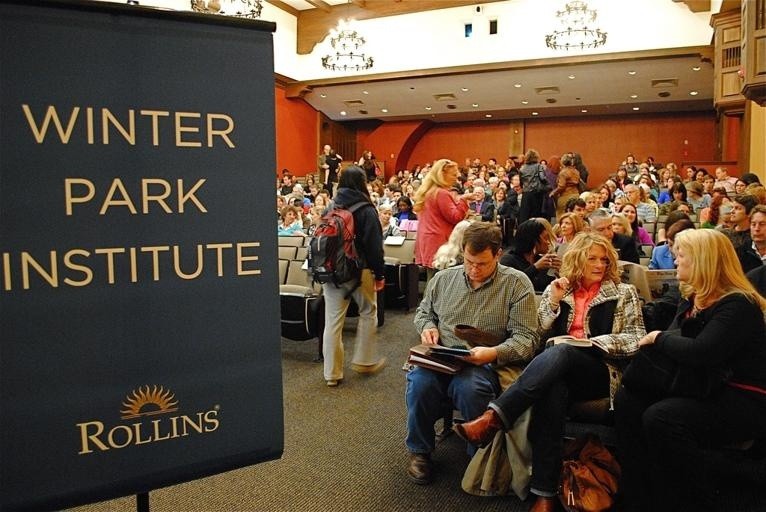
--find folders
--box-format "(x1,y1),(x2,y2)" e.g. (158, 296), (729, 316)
(406, 342), (465, 376)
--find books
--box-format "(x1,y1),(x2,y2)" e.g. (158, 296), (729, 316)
(546, 335), (592, 347)
(409, 341), (463, 374)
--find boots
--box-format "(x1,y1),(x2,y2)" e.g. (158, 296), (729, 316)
(454, 409), (500, 448)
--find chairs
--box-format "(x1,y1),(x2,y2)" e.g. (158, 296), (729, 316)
(276, 157), (417, 364)
(403, 164), (763, 511)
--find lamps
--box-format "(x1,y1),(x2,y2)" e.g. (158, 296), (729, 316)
(545, 1), (609, 52)
(321, 0), (374, 72)
(190, 0), (264, 19)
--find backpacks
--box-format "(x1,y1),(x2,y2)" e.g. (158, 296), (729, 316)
(306, 201), (376, 301)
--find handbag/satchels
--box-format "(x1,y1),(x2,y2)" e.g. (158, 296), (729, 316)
(529, 176), (552, 194)
(559, 460), (613, 512)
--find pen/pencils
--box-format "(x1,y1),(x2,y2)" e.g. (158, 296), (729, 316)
(554, 272), (561, 279)
(539, 253), (544, 257)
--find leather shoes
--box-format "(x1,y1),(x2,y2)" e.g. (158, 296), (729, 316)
(407, 453), (431, 485)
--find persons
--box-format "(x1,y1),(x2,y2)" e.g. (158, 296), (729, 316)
(456, 233), (650, 512)
(355, 148), (379, 183)
(404, 222), (539, 486)
(457, 148), (589, 292)
(369, 159), (479, 282)
(278, 168), (331, 237)
(551, 155), (766, 297)
(612, 229), (764, 511)
(303, 165), (384, 386)
(317, 145), (342, 196)
